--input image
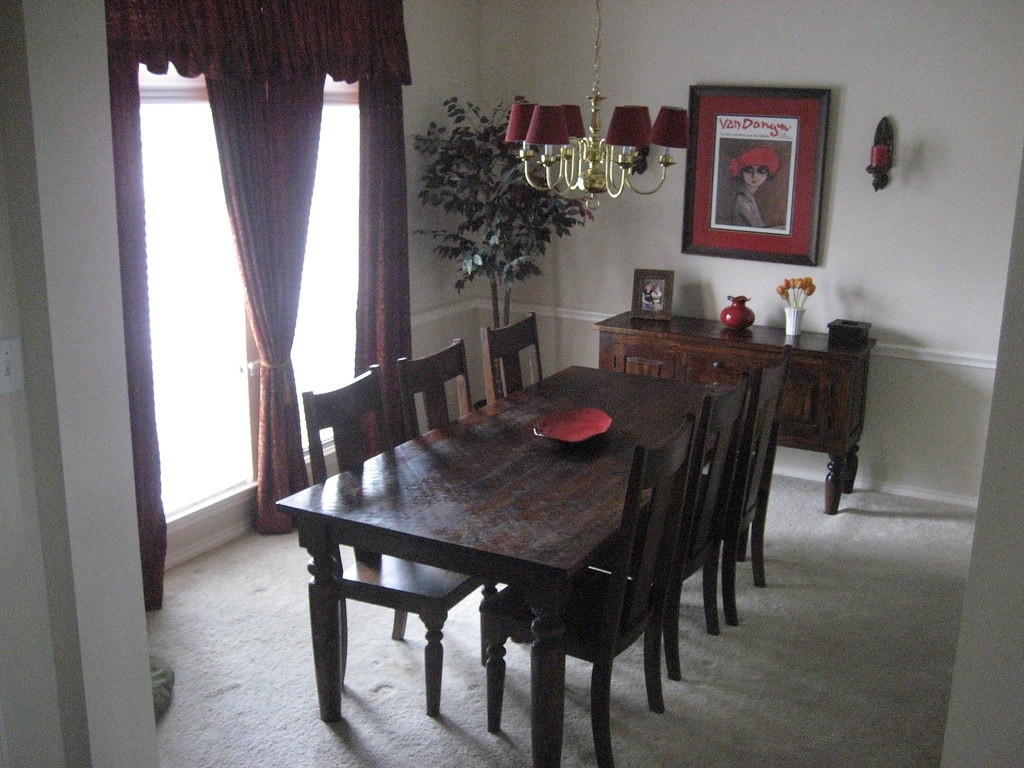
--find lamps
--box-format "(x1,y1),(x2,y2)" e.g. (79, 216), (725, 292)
(505, 0), (690, 210)
(865, 117), (893, 193)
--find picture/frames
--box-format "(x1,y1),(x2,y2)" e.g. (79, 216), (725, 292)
(681, 86), (831, 266)
(631, 269), (675, 321)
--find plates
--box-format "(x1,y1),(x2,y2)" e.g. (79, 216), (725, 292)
(533, 408), (614, 444)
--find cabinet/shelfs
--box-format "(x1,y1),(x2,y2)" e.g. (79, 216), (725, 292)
(593, 311), (876, 515)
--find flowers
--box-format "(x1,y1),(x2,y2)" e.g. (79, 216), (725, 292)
(776, 277), (816, 309)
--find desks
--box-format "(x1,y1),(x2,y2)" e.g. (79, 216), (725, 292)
(275, 365), (738, 768)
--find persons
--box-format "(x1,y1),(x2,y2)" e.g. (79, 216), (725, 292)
(642, 282), (663, 311)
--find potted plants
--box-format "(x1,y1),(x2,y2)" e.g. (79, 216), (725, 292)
(410, 96), (595, 414)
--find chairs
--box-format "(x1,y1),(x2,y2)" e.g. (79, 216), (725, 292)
(302, 312), (792, 768)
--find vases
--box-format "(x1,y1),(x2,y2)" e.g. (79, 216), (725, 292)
(784, 306), (805, 335)
(721, 296), (755, 329)
